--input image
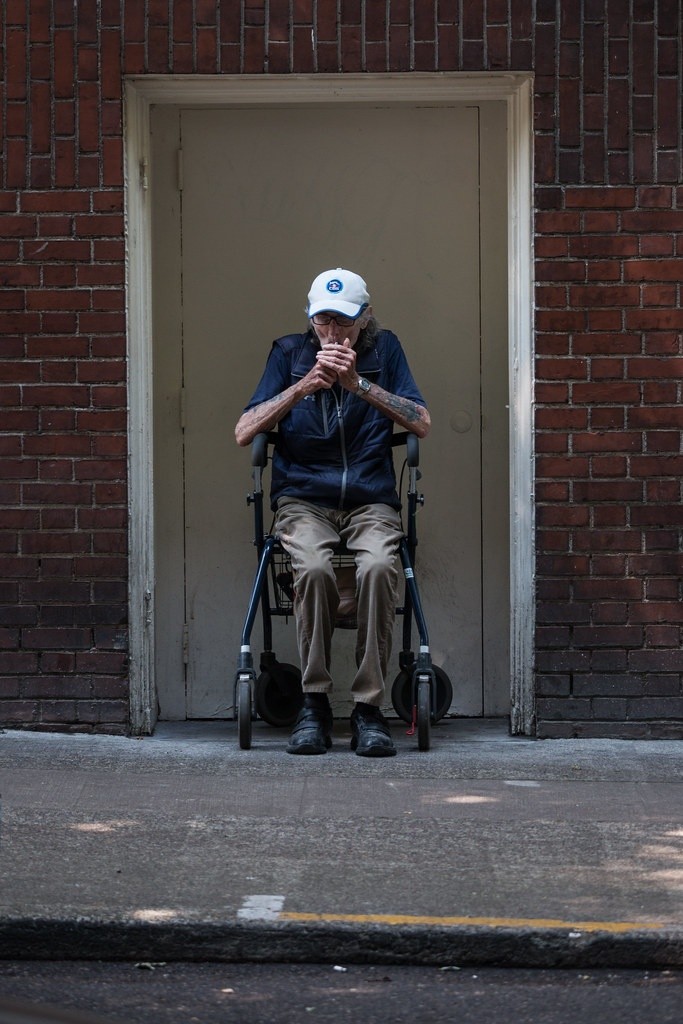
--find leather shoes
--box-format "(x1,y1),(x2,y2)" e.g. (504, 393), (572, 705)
(350, 708), (397, 756)
(287, 708), (333, 755)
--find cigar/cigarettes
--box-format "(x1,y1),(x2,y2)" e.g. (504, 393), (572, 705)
(332, 340), (344, 348)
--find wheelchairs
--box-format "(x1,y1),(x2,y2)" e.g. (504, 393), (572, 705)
(232, 431), (453, 752)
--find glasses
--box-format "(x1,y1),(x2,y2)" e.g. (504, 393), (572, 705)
(312, 307), (367, 327)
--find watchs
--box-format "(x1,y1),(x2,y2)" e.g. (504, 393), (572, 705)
(356, 374), (374, 401)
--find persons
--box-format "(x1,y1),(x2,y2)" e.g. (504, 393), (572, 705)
(228, 264), (459, 759)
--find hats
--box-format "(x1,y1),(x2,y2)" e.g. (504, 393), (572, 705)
(308, 267), (370, 318)
